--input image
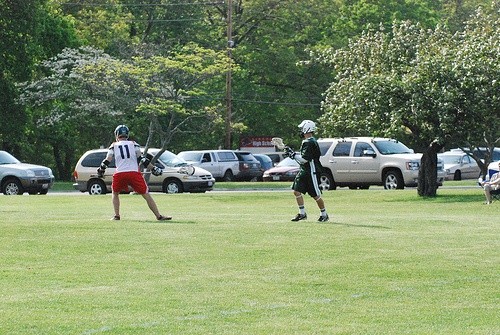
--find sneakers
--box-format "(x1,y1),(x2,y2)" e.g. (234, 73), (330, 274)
(291, 212), (307, 221)
(317, 213), (329, 222)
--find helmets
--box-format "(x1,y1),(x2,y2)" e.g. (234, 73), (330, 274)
(298, 120), (315, 134)
(114, 124), (129, 139)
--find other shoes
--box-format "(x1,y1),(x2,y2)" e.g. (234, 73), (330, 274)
(159, 215), (172, 220)
(112, 215), (120, 220)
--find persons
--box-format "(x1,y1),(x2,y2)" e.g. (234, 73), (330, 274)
(96, 125), (173, 221)
(283, 120), (329, 222)
(481, 161), (500, 205)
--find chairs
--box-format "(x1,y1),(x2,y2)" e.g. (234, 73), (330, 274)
(476, 169), (500, 203)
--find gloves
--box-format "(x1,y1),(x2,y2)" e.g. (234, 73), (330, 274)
(152, 166), (163, 177)
(283, 146), (296, 160)
(97, 168), (105, 179)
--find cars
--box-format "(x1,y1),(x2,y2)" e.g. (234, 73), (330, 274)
(0, 150), (55, 195)
(234, 151), (264, 181)
(294, 152), (304, 155)
(450, 147), (500, 164)
(262, 154), (301, 182)
(253, 154), (272, 171)
(437, 153), (485, 180)
(263, 153), (287, 167)
(485, 161), (500, 182)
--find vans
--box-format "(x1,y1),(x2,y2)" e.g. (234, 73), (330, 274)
(176, 150), (239, 182)
(72, 148), (216, 195)
(316, 137), (424, 191)
